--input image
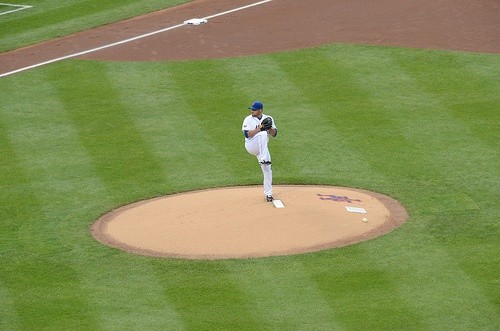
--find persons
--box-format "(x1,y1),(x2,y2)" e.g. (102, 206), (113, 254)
(242, 102), (277, 203)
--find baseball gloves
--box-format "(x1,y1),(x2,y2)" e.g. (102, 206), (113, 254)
(261, 116), (273, 131)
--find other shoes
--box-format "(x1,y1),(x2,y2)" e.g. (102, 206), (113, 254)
(266, 195), (273, 202)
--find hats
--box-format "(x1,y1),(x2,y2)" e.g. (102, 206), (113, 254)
(248, 102), (263, 110)
